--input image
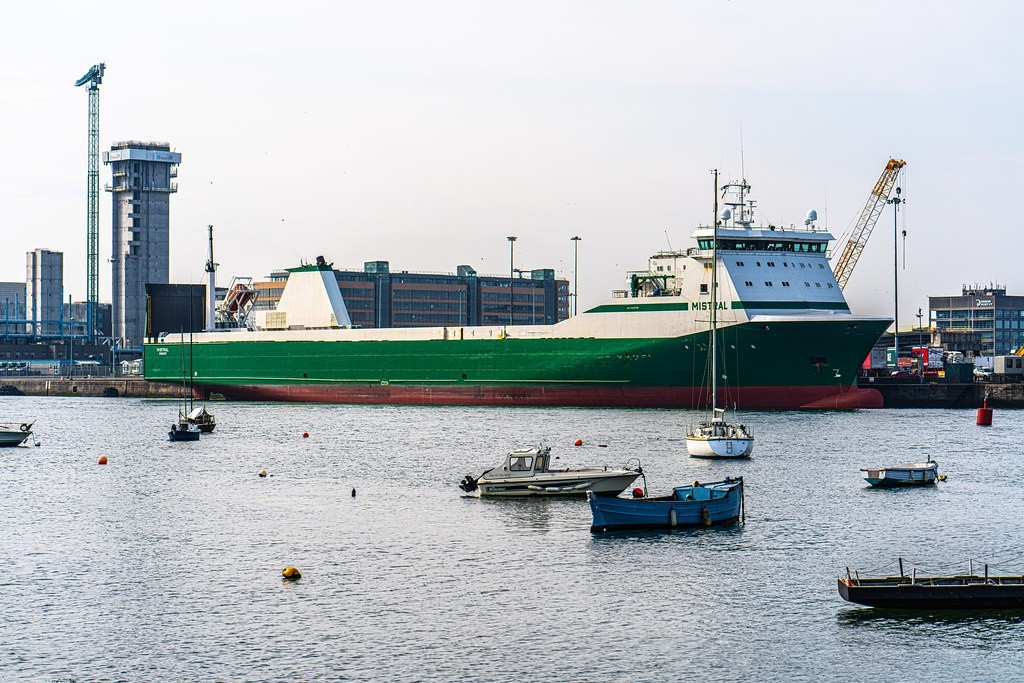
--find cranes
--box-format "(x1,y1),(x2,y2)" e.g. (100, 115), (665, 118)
(824, 157), (907, 294)
(73, 61), (107, 345)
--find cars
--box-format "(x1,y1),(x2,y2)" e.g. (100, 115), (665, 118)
(972, 367), (992, 381)
(891, 370), (918, 378)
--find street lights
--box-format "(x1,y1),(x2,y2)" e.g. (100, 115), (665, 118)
(506, 235), (518, 326)
(569, 236), (582, 317)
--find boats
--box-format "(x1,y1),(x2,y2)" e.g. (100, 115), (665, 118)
(585, 474), (745, 535)
(141, 124), (890, 413)
(860, 454), (948, 487)
(836, 557), (1024, 611)
(0, 419), (41, 448)
(458, 443), (649, 500)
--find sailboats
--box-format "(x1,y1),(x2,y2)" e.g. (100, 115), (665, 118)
(179, 284), (216, 432)
(167, 325), (201, 441)
(684, 167), (754, 458)
